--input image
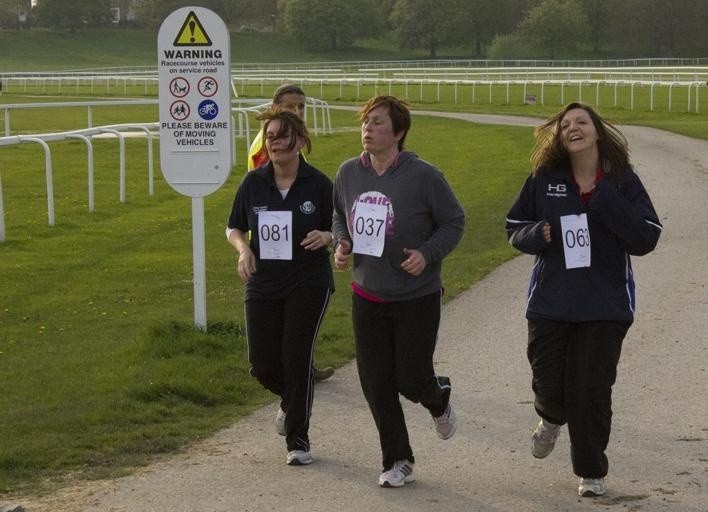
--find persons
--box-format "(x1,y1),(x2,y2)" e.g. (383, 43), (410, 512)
(331, 95), (465, 488)
(247, 84), (335, 382)
(225, 106), (334, 465)
(505, 100), (662, 497)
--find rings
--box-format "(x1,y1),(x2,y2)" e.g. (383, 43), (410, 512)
(407, 258), (412, 264)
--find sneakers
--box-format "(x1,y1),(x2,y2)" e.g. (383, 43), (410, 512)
(286, 450), (313, 465)
(577, 476), (606, 497)
(379, 459), (416, 488)
(432, 400), (456, 441)
(530, 417), (561, 458)
(274, 407), (287, 435)
